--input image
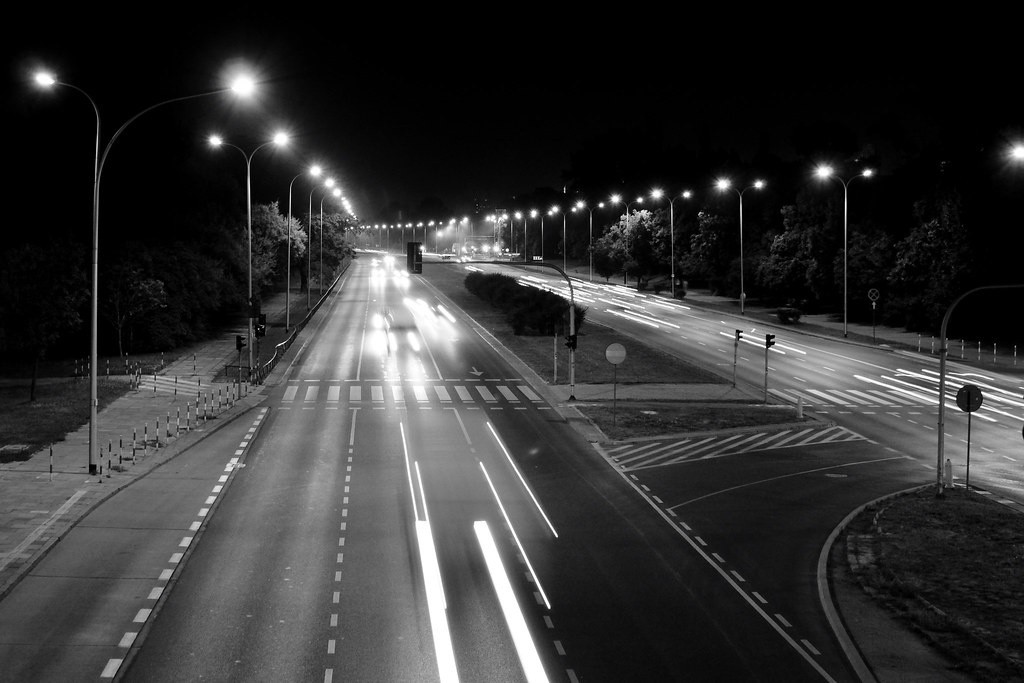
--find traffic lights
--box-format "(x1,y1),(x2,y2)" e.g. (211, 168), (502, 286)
(565, 334), (575, 350)
(765, 333), (778, 348)
(736, 329), (744, 341)
(237, 335), (246, 350)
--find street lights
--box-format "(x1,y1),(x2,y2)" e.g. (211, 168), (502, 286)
(317, 189), (343, 300)
(531, 210), (555, 273)
(815, 165), (874, 337)
(25, 60), (258, 476)
(285, 166), (321, 332)
(552, 205), (578, 275)
(502, 212), (516, 262)
(651, 188), (692, 298)
(611, 194), (644, 286)
(346, 214), (499, 257)
(716, 178), (767, 315)
(205, 129), (292, 378)
(515, 210), (538, 271)
(305, 177), (335, 312)
(577, 200), (605, 283)
